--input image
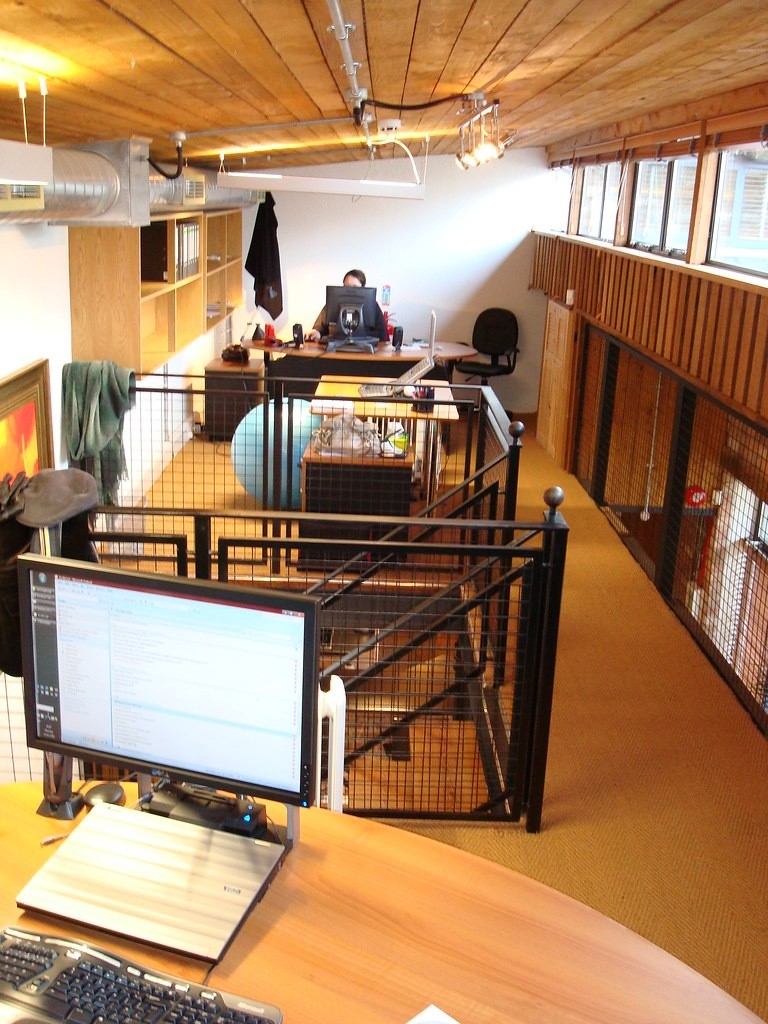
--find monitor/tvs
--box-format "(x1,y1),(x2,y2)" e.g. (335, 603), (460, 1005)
(326, 286), (377, 354)
(17, 553), (320, 836)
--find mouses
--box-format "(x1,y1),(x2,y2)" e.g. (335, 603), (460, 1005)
(306, 336), (315, 342)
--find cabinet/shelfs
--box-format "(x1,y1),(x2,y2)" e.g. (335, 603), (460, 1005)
(65, 208), (245, 379)
(206, 356), (265, 442)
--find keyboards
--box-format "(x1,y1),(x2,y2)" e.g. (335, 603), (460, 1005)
(319, 338), (379, 347)
(0, 926), (282, 1024)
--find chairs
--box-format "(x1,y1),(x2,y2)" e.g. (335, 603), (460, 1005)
(454, 306), (521, 421)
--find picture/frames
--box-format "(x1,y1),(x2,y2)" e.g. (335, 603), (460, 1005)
(0, 358), (56, 486)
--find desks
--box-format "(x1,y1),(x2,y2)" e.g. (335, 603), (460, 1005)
(308, 372), (459, 530)
(242, 335), (478, 442)
(0, 776), (768, 1024)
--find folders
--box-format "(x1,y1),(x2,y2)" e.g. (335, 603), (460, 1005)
(141, 222), (199, 283)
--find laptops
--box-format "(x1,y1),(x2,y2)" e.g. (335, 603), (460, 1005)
(395, 311), (437, 393)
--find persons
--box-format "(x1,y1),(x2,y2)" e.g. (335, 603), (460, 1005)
(302, 269), (390, 341)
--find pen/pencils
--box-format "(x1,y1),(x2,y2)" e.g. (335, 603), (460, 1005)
(415, 386), (434, 412)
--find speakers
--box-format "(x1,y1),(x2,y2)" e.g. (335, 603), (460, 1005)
(392, 327), (403, 351)
(293, 324), (303, 349)
